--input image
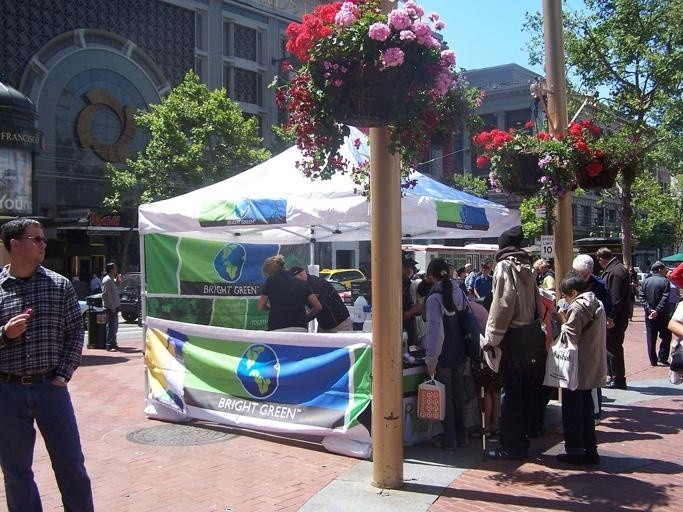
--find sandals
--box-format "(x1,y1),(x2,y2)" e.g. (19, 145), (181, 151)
(486, 447), (524, 460)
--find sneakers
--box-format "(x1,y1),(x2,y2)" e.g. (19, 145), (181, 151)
(556, 448), (599, 465)
(432, 429), (499, 450)
(602, 378), (626, 389)
(651, 357), (670, 366)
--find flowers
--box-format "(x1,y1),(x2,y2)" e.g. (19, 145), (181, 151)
(271, 1), (485, 199)
(473, 120), (550, 197)
(520, 115), (628, 237)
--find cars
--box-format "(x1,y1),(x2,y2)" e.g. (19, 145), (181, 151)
(319, 269), (366, 290)
(78, 300), (107, 329)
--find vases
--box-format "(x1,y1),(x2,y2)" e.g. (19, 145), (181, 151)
(514, 157), (538, 190)
(576, 164), (617, 188)
(340, 81), (384, 126)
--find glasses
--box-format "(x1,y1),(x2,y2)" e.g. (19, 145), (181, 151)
(27, 236), (48, 245)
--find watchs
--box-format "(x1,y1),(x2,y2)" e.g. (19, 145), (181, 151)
(56, 375), (67, 385)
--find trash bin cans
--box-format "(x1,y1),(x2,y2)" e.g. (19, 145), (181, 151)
(86, 293), (106, 349)
(351, 280), (371, 306)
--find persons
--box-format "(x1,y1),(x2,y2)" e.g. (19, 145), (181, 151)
(639, 261), (683, 368)
(89, 272), (101, 294)
(101, 263), (123, 352)
(597, 247), (633, 390)
(257, 254), (323, 333)
(402, 225), (608, 466)
(289, 265), (353, 333)
(0, 218), (95, 512)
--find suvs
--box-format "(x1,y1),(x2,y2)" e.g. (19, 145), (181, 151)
(87, 272), (141, 321)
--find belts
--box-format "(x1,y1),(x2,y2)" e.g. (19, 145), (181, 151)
(0, 368), (57, 384)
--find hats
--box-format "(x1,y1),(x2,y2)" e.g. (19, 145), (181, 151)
(498, 225), (523, 249)
(289, 266), (304, 276)
(402, 249), (419, 266)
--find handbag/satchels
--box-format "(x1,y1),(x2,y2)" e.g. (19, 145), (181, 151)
(462, 313), (483, 356)
(542, 329), (578, 391)
(497, 321), (548, 382)
